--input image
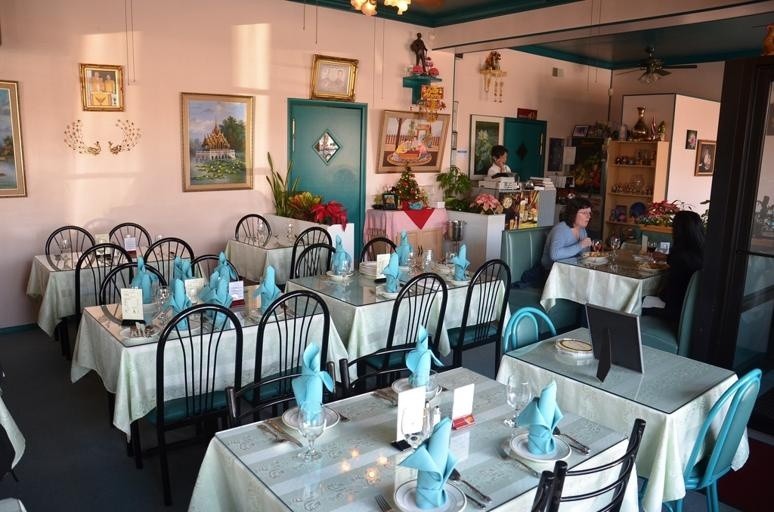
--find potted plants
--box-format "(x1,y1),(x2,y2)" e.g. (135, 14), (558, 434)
(434, 166), (472, 210)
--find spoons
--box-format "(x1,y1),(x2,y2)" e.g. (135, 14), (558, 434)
(450, 469), (492, 504)
(552, 427), (591, 451)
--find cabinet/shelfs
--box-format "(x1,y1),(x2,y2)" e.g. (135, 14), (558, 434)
(367, 208), (447, 265)
(602, 140), (670, 253)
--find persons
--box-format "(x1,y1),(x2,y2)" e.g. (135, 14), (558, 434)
(642, 211), (705, 325)
(487, 145), (512, 177)
(90, 72), (116, 94)
(410, 33), (427, 72)
(520, 197), (602, 291)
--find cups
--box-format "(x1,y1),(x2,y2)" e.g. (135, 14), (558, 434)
(408, 369), (438, 402)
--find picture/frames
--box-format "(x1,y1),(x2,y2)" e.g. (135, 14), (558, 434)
(376, 110), (451, 174)
(572, 125), (590, 137)
(694, 139), (716, 176)
(469, 114), (505, 181)
(79, 63), (125, 111)
(0, 81), (28, 197)
(180, 92), (254, 192)
(382, 194), (398, 210)
(310, 54), (359, 103)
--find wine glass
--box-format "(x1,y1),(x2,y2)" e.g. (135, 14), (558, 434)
(53, 222), (474, 338)
(296, 402), (327, 459)
(503, 375), (531, 427)
(583, 237), (667, 273)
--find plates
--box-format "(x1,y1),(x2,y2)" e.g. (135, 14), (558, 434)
(392, 478), (466, 511)
(510, 432), (571, 463)
(281, 404), (347, 431)
(390, 375), (442, 396)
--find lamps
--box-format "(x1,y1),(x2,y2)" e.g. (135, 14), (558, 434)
(350, 0), (412, 16)
(646, 67), (658, 88)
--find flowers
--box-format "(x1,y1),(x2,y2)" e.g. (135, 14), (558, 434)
(644, 200), (680, 217)
(288, 192), (348, 232)
(470, 193), (504, 216)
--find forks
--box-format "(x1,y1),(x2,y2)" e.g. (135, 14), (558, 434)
(495, 445), (544, 481)
(374, 494), (393, 512)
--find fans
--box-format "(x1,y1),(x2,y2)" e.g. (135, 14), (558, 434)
(613, 29), (698, 76)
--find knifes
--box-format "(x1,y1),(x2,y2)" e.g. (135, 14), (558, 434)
(265, 419), (303, 449)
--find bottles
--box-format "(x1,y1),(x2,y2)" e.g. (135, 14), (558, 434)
(608, 150), (656, 225)
(629, 106), (649, 138)
(420, 400), (440, 436)
